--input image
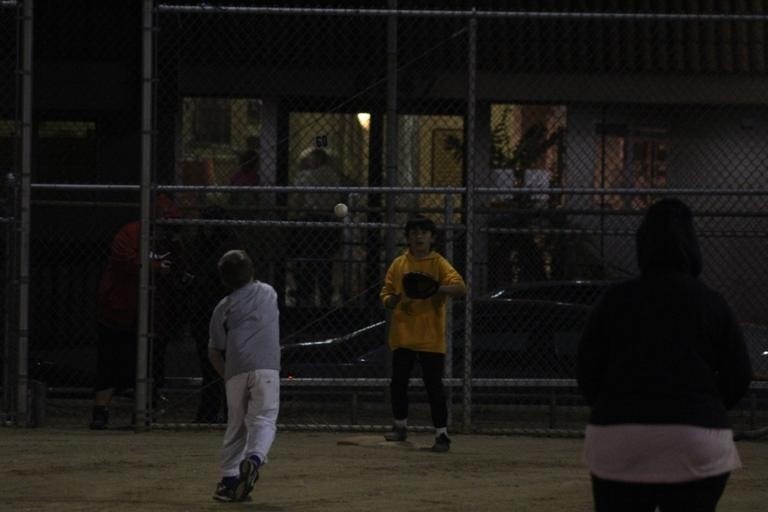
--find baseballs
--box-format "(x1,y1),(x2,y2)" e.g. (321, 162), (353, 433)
(334, 204), (348, 218)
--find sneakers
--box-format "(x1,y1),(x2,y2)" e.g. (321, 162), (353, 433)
(230, 456), (259, 502)
(89, 404), (114, 431)
(431, 432), (452, 453)
(212, 481), (257, 505)
(383, 423), (407, 442)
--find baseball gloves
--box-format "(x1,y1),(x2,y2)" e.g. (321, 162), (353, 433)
(401, 271), (441, 300)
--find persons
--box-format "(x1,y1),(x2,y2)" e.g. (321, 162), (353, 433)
(170, 204), (243, 424)
(561, 196), (753, 512)
(223, 146), (260, 207)
(207, 249), (283, 504)
(87, 193), (182, 431)
(379, 214), (467, 450)
(286, 148), (343, 310)
